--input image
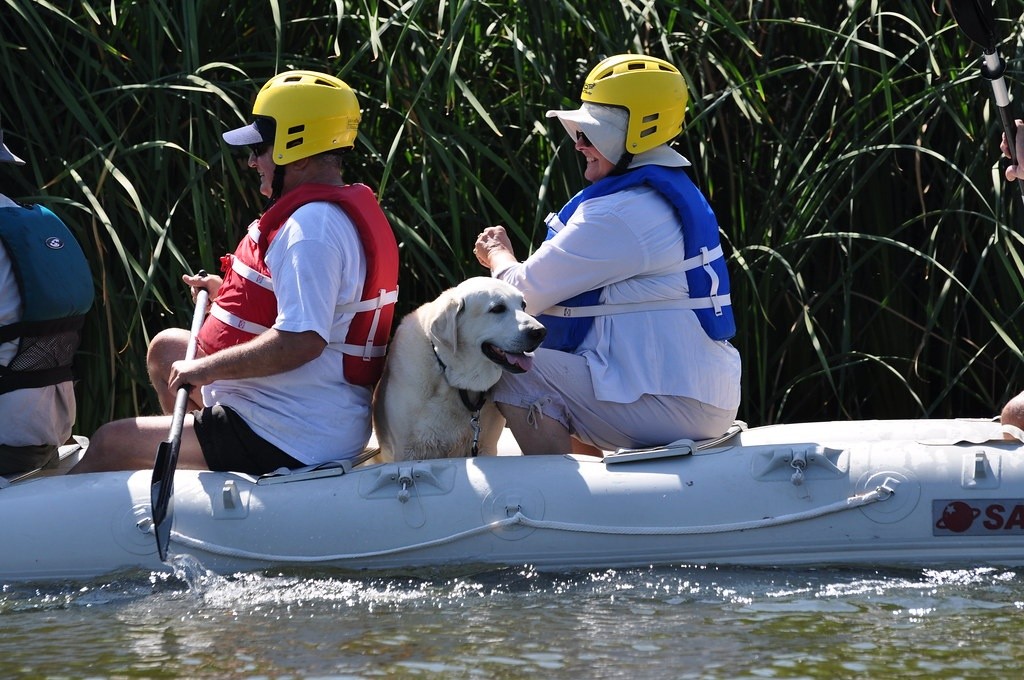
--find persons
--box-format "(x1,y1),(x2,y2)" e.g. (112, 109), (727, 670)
(472, 53), (740, 454)
(1000, 119), (1024, 440)
(0, 138), (76, 484)
(68, 69), (398, 475)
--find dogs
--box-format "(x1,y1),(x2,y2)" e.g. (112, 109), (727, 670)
(370, 276), (548, 464)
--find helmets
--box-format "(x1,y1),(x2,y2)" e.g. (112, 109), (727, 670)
(252, 70), (363, 166)
(581, 55), (689, 155)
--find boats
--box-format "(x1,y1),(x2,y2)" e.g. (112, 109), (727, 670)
(0, 416), (1024, 593)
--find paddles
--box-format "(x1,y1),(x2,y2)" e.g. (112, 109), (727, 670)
(150, 267), (208, 561)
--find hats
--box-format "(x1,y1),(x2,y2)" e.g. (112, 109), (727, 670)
(545, 102), (692, 168)
(0, 130), (25, 166)
(222, 122), (265, 146)
